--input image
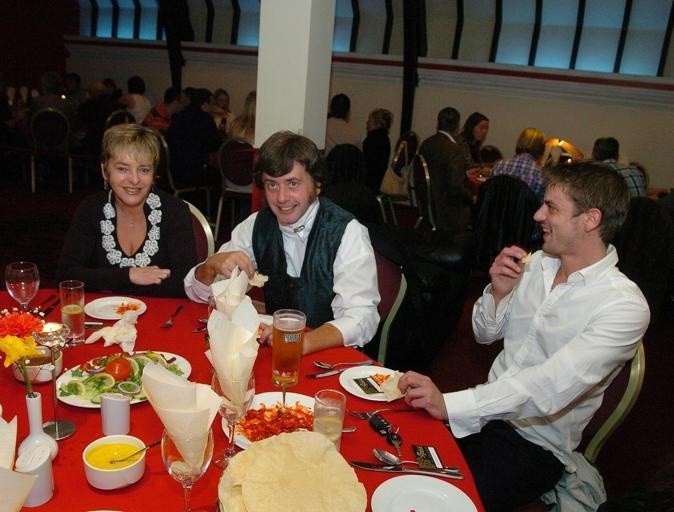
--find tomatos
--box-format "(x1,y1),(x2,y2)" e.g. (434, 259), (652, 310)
(106, 356), (129, 380)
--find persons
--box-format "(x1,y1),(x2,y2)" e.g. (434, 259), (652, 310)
(398, 159), (652, 512)
(183, 128), (379, 358)
(29, 71), (256, 190)
(316, 143), (398, 262)
(326, 93), (650, 205)
(54, 123), (194, 296)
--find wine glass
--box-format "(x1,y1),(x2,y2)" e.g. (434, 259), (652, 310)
(211, 368), (255, 469)
(158, 424), (215, 511)
(216, 390), (343, 452)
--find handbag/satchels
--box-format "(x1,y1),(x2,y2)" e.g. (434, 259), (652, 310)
(380, 167), (409, 196)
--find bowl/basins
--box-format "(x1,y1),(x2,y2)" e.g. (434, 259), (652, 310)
(81, 435), (148, 491)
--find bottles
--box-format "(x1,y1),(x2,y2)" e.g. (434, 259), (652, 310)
(96, 393), (132, 436)
(14, 444), (53, 508)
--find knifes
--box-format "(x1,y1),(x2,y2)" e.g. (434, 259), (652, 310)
(306, 368), (348, 380)
(342, 457), (470, 479)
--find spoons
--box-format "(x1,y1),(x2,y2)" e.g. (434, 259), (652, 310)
(366, 446), (465, 473)
(314, 358), (377, 370)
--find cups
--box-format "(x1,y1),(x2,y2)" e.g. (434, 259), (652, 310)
(271, 308), (304, 387)
(58, 280), (85, 344)
(313, 390), (346, 454)
(3, 262), (39, 309)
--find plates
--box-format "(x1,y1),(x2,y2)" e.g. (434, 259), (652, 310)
(370, 475), (479, 512)
(82, 296), (149, 322)
(339, 365), (404, 403)
(50, 349), (193, 411)
